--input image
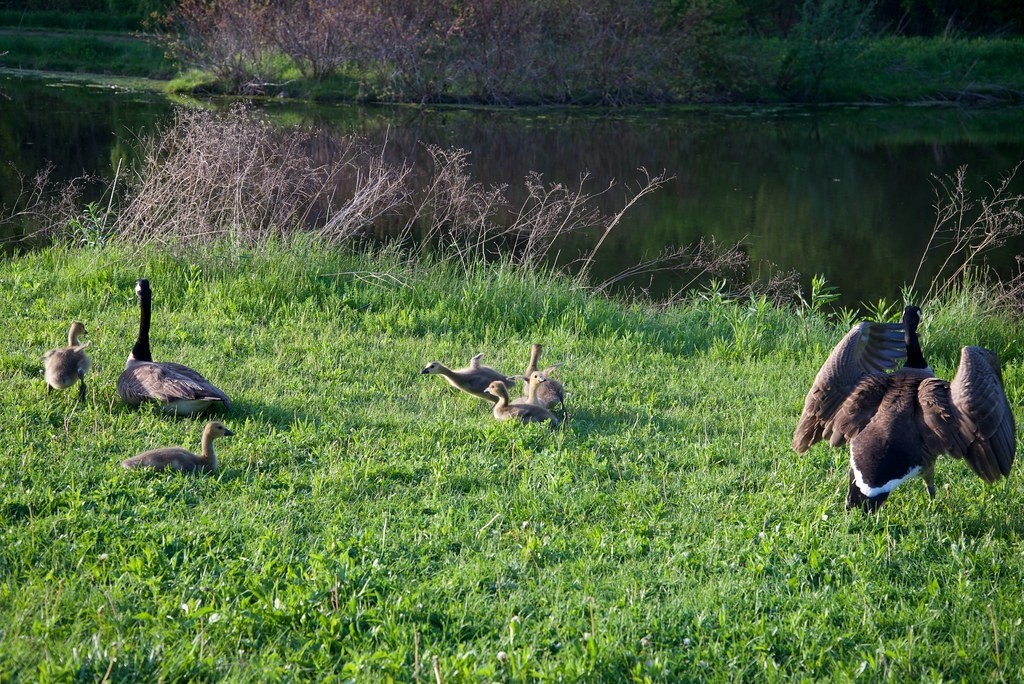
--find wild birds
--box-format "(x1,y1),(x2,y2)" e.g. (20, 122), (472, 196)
(39, 321), (93, 402)
(116, 278), (231, 415)
(121, 421), (236, 474)
(792, 305), (1017, 515)
(420, 343), (565, 422)
